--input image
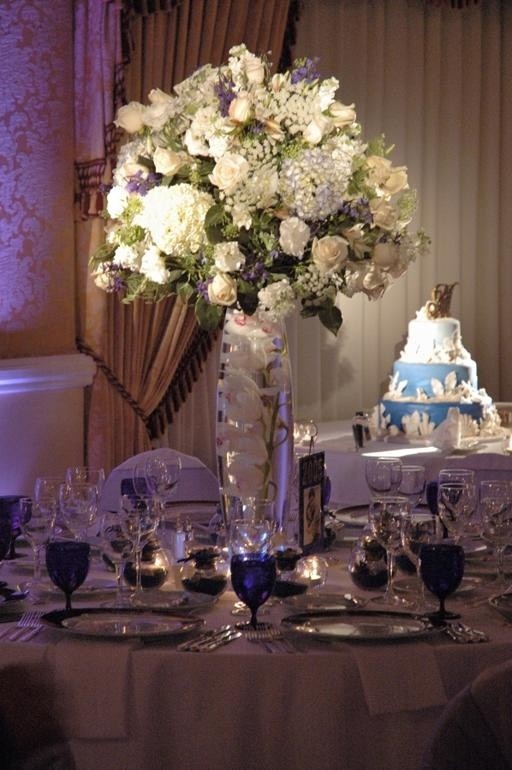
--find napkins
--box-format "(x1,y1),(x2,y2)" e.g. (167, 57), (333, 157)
(331, 639), (449, 717)
(46, 631), (146, 742)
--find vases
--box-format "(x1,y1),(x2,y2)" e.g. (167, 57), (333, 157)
(209, 308), (299, 554)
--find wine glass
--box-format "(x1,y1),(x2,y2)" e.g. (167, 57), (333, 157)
(364, 458), (511, 618)
(0, 455), (181, 613)
(229, 498), (303, 631)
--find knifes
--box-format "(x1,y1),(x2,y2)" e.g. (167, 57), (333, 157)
(437, 620), (491, 643)
(175, 624), (242, 653)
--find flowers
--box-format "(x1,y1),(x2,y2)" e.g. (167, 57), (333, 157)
(89, 34), (428, 337)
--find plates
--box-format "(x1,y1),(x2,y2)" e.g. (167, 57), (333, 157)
(334, 502), (443, 528)
(39, 607), (205, 641)
(280, 609), (435, 643)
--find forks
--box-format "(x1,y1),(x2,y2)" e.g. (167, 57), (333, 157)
(1, 609), (47, 643)
(243, 622), (298, 655)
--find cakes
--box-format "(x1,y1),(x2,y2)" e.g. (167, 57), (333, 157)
(370, 282), (504, 440)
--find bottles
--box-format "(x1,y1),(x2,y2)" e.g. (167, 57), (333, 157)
(353, 410), (367, 450)
(180, 544), (229, 605)
(348, 535), (392, 591)
(295, 555), (328, 593)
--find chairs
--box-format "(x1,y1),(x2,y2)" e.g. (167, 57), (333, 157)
(457, 451), (509, 498)
(422, 656), (509, 767)
(101, 448), (222, 508)
